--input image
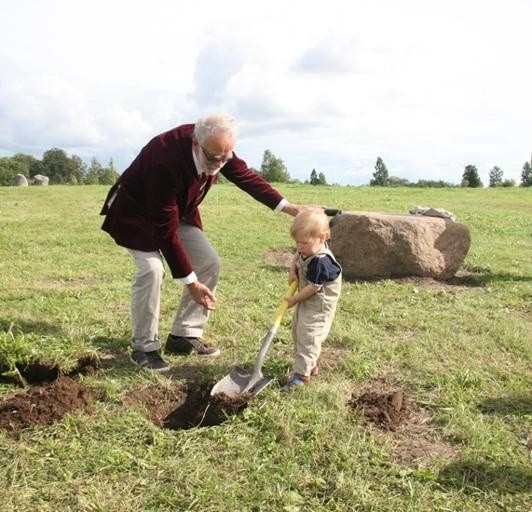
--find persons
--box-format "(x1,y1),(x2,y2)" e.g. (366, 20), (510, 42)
(98, 112), (327, 373)
(278, 210), (343, 390)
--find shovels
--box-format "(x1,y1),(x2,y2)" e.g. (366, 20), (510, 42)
(209, 280), (298, 408)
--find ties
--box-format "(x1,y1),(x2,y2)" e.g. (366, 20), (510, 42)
(193, 171), (209, 206)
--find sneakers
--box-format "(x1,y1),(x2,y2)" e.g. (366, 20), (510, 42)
(130, 352), (171, 373)
(282, 373), (311, 392)
(289, 367), (319, 376)
(164, 334), (221, 358)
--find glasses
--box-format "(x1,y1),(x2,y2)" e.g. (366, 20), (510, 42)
(202, 148), (233, 162)
